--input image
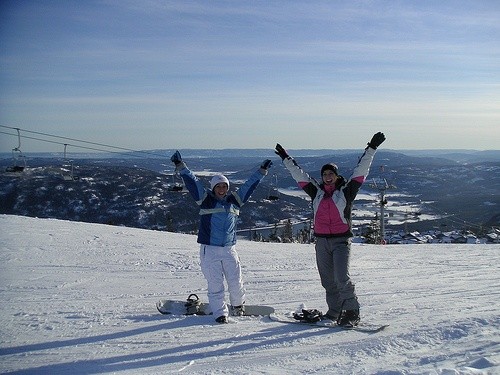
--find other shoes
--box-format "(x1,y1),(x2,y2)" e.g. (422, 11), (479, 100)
(233, 305), (244, 316)
(215, 315), (228, 324)
(322, 314), (339, 321)
(338, 313), (360, 328)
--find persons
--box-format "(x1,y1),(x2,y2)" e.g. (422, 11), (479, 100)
(275, 132), (386, 328)
(170, 150), (273, 323)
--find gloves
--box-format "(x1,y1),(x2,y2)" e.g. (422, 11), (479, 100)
(259, 159), (273, 175)
(171, 150), (186, 171)
(367, 132), (386, 151)
(274, 143), (289, 162)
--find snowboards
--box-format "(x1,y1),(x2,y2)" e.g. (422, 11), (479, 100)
(155, 298), (275, 317)
(268, 312), (390, 333)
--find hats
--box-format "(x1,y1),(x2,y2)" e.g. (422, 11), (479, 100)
(210, 175), (229, 192)
(321, 163), (339, 178)
